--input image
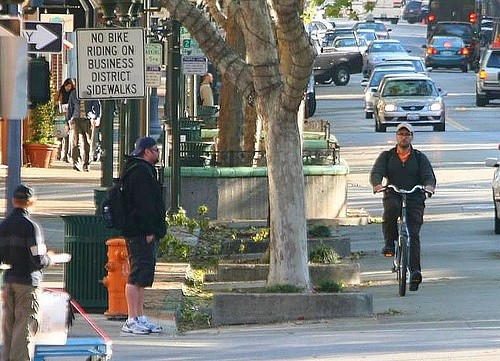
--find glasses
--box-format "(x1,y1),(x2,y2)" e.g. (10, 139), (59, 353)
(149, 147), (159, 152)
(398, 133), (411, 136)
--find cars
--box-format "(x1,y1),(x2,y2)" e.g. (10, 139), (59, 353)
(475, 47), (500, 107)
(361, 61), (417, 92)
(381, 57), (433, 78)
(362, 41), (411, 75)
(484, 145), (500, 236)
(320, 19), (360, 47)
(421, 36), (470, 74)
(401, 0), (429, 24)
(354, 20), (393, 39)
(371, 77), (448, 133)
(360, 66), (417, 120)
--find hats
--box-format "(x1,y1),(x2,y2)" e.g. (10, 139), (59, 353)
(130, 137), (157, 156)
(13, 185), (34, 198)
(395, 123), (413, 133)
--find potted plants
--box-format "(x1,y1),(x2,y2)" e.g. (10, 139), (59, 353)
(22, 106), (53, 169)
(42, 102), (61, 169)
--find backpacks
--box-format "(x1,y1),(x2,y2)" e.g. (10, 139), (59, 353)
(101, 161), (152, 228)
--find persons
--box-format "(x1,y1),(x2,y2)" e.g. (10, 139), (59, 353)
(58, 78), (75, 163)
(0, 184), (54, 361)
(118, 137), (167, 334)
(199, 74), (216, 108)
(369, 122), (437, 290)
(65, 87), (102, 172)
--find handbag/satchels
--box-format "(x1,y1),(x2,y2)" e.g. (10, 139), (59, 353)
(54, 116), (68, 138)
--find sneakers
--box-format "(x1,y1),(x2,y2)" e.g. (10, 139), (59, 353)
(382, 246), (396, 254)
(409, 270), (421, 291)
(122, 320), (151, 334)
(139, 317), (162, 332)
(73, 164), (81, 171)
(84, 168), (90, 172)
(57, 156), (61, 160)
(63, 156), (68, 163)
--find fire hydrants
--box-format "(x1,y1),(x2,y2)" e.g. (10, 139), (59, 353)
(96, 238), (132, 321)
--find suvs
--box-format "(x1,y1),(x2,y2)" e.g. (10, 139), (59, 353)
(426, 21), (478, 63)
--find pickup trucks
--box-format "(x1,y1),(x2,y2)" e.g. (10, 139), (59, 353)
(312, 48), (363, 86)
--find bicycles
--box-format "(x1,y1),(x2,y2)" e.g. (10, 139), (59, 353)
(372, 184), (434, 298)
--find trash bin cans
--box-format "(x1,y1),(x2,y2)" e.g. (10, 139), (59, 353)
(60, 213), (124, 313)
(197, 106), (216, 127)
(94, 186), (109, 213)
(179, 118), (202, 160)
